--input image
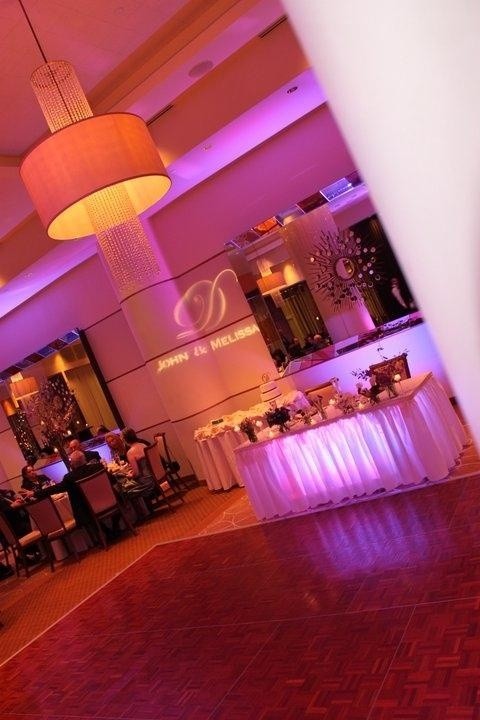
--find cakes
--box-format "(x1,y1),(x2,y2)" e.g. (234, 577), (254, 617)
(259, 374), (285, 409)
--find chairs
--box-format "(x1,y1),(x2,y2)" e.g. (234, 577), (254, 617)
(0, 432), (190, 579)
(370, 353), (411, 383)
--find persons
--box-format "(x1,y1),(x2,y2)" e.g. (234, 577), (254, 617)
(112, 427), (158, 528)
(27, 450), (124, 549)
(104, 431), (130, 466)
(69, 438), (101, 473)
(0, 488), (48, 565)
(19, 465), (54, 499)
(271, 328), (332, 373)
(371, 234), (419, 322)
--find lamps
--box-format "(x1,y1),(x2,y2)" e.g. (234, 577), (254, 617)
(251, 241), (288, 309)
(17, 0), (173, 291)
(9, 372), (41, 406)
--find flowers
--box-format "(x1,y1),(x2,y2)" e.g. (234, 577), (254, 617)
(233, 343), (405, 444)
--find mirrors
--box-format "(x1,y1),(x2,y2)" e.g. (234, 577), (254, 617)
(303, 225), (389, 314)
